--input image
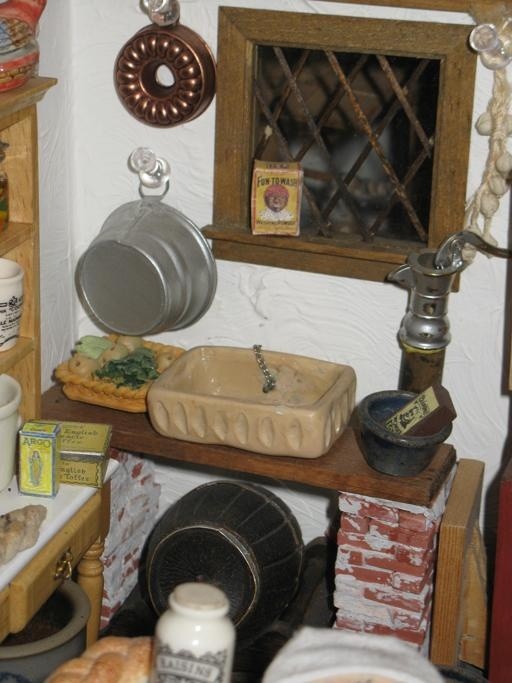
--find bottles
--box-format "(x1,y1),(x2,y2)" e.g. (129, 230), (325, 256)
(153, 582), (236, 677)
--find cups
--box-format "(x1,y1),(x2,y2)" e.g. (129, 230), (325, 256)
(0, 257), (25, 354)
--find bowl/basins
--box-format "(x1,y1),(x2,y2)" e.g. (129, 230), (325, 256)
(1, 372), (23, 492)
(143, 478), (307, 644)
(356, 389), (454, 478)
(74, 202), (217, 338)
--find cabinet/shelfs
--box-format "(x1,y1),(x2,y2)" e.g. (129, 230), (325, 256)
(1, 76), (122, 654)
